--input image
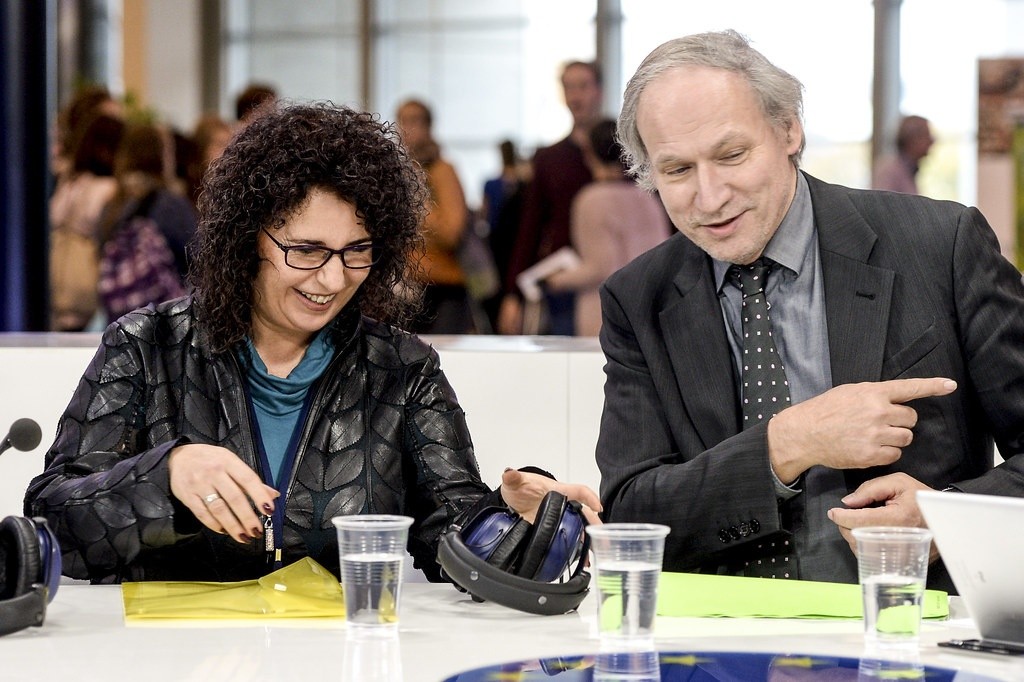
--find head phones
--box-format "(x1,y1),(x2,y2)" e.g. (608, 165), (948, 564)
(0, 515), (62, 636)
(436, 491), (592, 615)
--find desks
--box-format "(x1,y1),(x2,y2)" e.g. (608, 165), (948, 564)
(0, 557), (1024, 682)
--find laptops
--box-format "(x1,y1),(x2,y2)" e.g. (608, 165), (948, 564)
(913, 489), (1024, 654)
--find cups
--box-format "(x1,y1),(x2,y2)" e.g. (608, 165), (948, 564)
(592, 642), (661, 682)
(586, 523), (671, 641)
(850, 526), (934, 633)
(343, 628), (402, 680)
(331, 514), (415, 627)
(856, 634), (926, 682)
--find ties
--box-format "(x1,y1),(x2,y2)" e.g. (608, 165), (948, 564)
(727, 262), (792, 580)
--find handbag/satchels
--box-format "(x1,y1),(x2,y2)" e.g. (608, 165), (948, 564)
(48, 209), (101, 332)
(93, 194), (185, 323)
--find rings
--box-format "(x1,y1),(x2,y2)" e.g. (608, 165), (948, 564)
(206, 494), (219, 505)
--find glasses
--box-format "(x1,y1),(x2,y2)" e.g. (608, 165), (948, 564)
(262, 227), (382, 271)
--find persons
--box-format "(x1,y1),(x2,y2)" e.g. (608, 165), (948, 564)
(881, 115), (933, 195)
(38, 59), (679, 334)
(595, 30), (1024, 596)
(21, 95), (605, 585)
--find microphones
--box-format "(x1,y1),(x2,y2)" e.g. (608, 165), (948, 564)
(0, 418), (42, 456)
